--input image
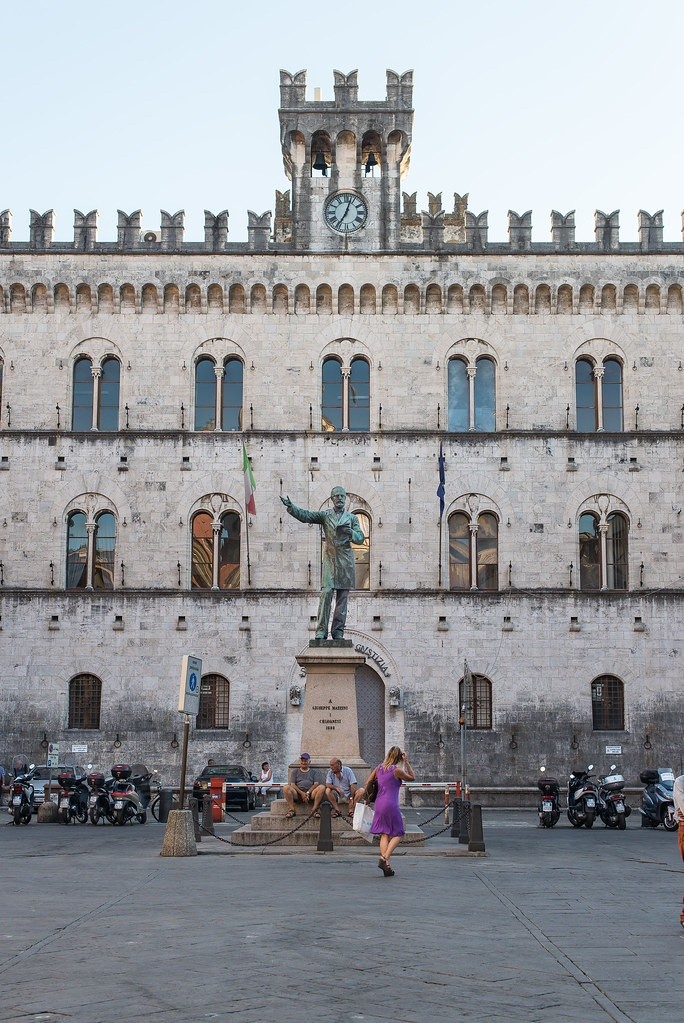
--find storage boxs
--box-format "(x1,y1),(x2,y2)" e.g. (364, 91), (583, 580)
(639, 769), (659, 785)
(111, 763), (132, 780)
(87, 772), (104, 787)
(58, 773), (76, 786)
(537, 777), (559, 791)
(603, 775), (625, 790)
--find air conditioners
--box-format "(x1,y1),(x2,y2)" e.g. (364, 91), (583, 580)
(139, 229), (161, 242)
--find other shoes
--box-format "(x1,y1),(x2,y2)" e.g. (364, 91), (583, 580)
(680, 897), (684, 927)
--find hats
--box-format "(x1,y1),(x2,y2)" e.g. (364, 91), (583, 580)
(300, 752), (310, 760)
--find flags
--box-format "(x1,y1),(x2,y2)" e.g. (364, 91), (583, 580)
(242, 444), (257, 515)
(436, 444), (445, 515)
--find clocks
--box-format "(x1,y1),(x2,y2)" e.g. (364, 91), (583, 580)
(323, 189), (369, 236)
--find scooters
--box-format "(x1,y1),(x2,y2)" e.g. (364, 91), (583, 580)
(7, 775), (35, 826)
(567, 764), (601, 828)
(85, 771), (117, 823)
(55, 771), (89, 823)
(104, 764), (143, 824)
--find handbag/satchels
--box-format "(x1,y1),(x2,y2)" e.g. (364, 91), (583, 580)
(363, 774), (379, 803)
(353, 800), (376, 843)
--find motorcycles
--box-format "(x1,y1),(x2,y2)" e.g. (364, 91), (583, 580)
(595, 763), (631, 830)
(533, 766), (563, 828)
(641, 768), (678, 832)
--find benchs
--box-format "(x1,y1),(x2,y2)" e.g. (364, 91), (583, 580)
(409, 787), (645, 808)
(0, 786), (282, 807)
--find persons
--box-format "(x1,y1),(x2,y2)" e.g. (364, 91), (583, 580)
(325, 757), (365, 818)
(363, 746), (415, 877)
(255, 762), (273, 807)
(673, 776), (684, 927)
(283, 752), (325, 818)
(279, 486), (365, 640)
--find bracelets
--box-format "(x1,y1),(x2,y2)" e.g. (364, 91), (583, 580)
(307, 791), (310, 795)
(350, 797), (353, 800)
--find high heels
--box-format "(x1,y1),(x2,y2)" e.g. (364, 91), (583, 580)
(378, 856), (393, 874)
(384, 870), (394, 877)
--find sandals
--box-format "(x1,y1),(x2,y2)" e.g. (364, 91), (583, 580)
(310, 810), (321, 818)
(285, 810), (296, 818)
(332, 810), (342, 818)
(347, 812), (354, 818)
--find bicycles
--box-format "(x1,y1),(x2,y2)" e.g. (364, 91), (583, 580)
(149, 776), (180, 822)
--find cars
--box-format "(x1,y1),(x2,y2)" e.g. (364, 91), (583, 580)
(192, 766), (253, 813)
(23, 765), (87, 813)
